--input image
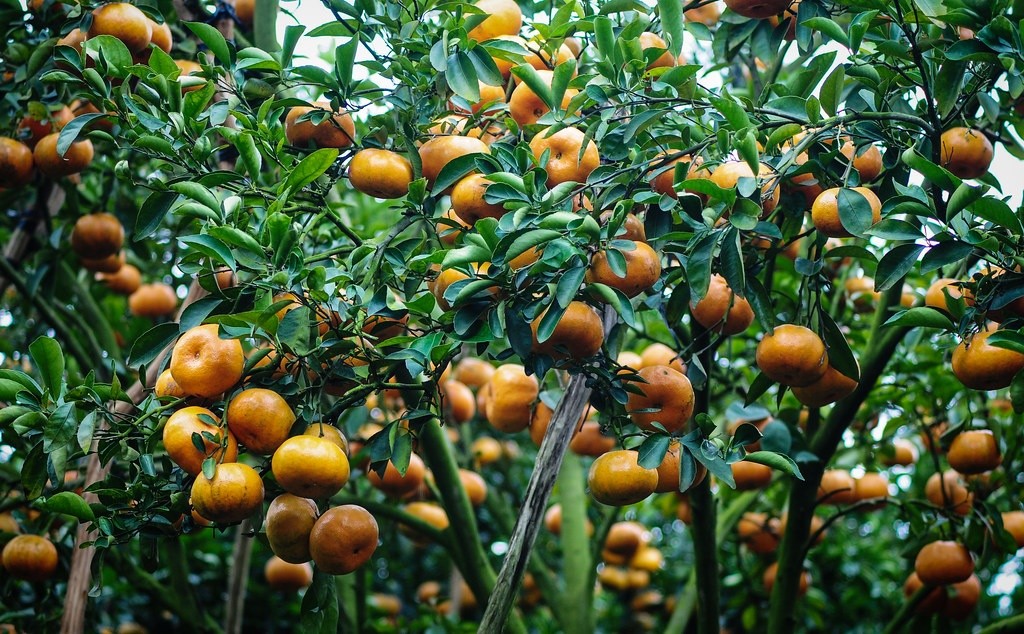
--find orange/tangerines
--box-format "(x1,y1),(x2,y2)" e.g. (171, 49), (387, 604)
(0, 0), (1024, 634)
